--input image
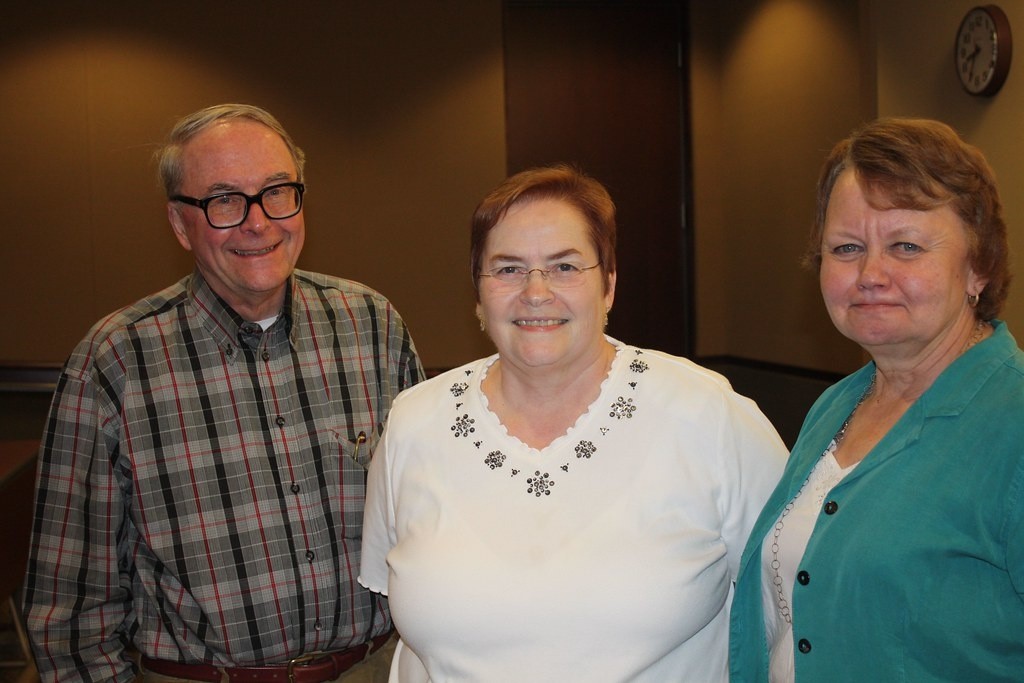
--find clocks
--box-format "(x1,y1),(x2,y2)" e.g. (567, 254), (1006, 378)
(954, 3), (1013, 98)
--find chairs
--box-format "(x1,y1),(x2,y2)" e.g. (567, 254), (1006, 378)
(0, 439), (40, 667)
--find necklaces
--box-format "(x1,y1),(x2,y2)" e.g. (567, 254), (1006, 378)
(770, 319), (986, 626)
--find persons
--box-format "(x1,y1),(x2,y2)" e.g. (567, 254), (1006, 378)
(22, 104), (428, 683)
(728, 117), (1024, 683)
(356, 164), (791, 683)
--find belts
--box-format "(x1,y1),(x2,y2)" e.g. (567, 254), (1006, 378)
(141, 627), (396, 683)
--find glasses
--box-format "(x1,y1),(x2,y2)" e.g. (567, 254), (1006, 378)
(169, 182), (305, 229)
(478, 261), (602, 289)
